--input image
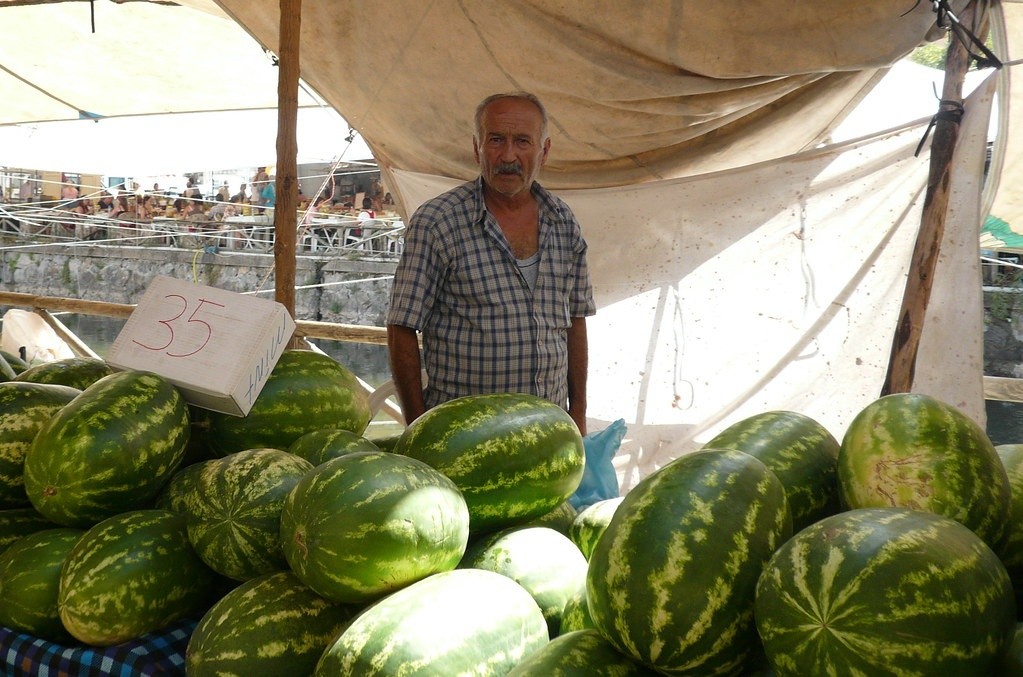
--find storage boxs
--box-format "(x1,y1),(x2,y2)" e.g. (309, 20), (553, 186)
(105, 274), (297, 418)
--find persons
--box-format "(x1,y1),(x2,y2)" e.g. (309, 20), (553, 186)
(61, 166), (392, 235)
(386, 89), (598, 440)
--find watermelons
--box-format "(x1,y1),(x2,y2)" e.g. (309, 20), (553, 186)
(0, 347), (591, 677)
(507, 393), (1023, 677)
